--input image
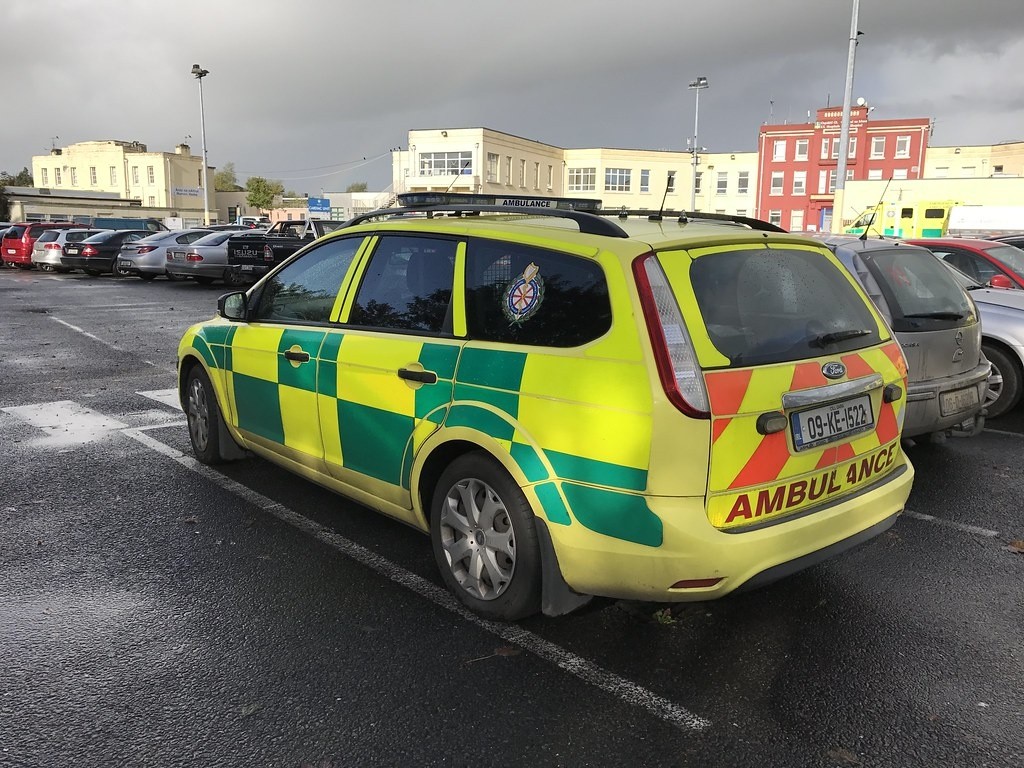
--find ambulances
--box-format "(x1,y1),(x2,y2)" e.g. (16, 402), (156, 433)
(171, 191), (916, 625)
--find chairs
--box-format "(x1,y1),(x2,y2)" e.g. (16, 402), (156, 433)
(406, 251), (453, 329)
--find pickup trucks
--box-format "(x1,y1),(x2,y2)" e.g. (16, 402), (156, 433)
(223, 217), (363, 290)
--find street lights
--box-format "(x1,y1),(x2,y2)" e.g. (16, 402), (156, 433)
(191, 63), (212, 230)
(686, 75), (707, 211)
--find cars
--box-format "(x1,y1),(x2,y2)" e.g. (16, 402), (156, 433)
(0, 215), (280, 287)
(730, 231), (1024, 453)
